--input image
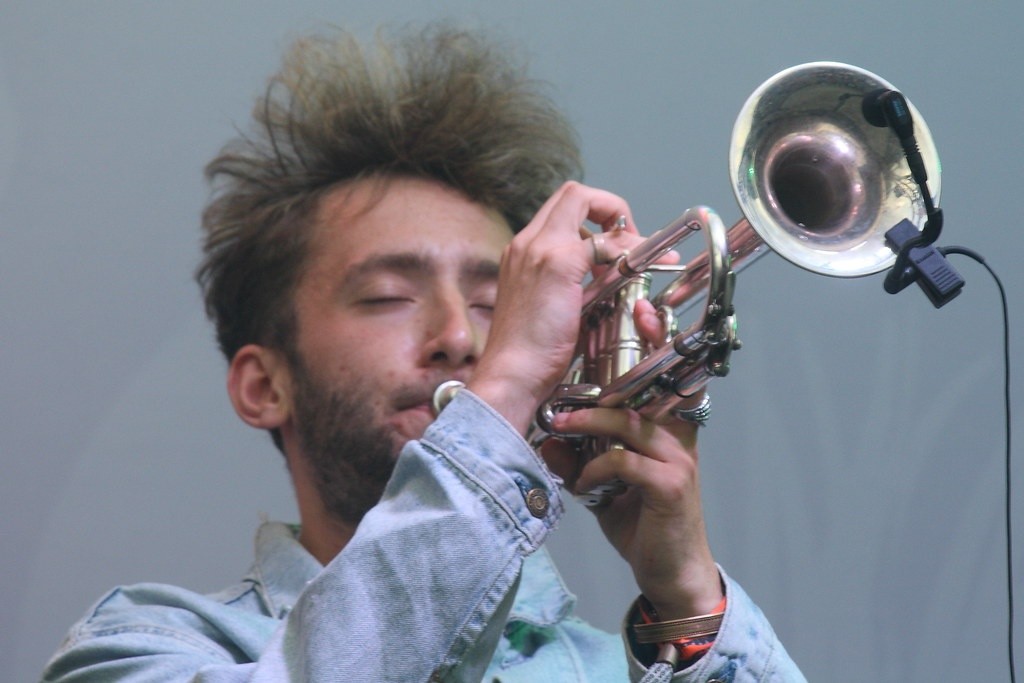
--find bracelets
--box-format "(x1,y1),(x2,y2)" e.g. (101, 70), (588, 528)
(630, 610), (726, 645)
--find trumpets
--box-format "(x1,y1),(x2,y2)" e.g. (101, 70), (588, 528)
(431, 62), (944, 510)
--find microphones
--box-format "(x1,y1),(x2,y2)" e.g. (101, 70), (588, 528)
(862, 88), (927, 184)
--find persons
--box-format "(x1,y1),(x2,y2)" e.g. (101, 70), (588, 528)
(42, 21), (813, 683)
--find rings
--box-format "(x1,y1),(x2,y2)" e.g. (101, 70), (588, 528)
(662, 393), (713, 427)
(591, 230), (609, 267)
